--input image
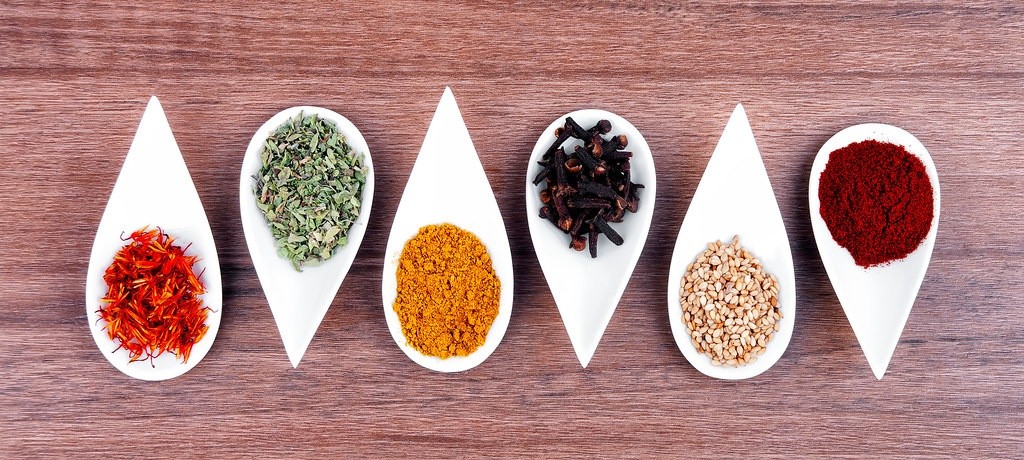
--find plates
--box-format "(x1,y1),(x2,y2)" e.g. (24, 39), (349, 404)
(808, 123), (941, 377)
(526, 106), (657, 368)
(85, 95), (223, 381)
(381, 86), (515, 373)
(240, 106), (374, 366)
(666, 103), (796, 380)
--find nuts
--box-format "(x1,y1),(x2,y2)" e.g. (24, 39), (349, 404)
(679, 234), (785, 368)
